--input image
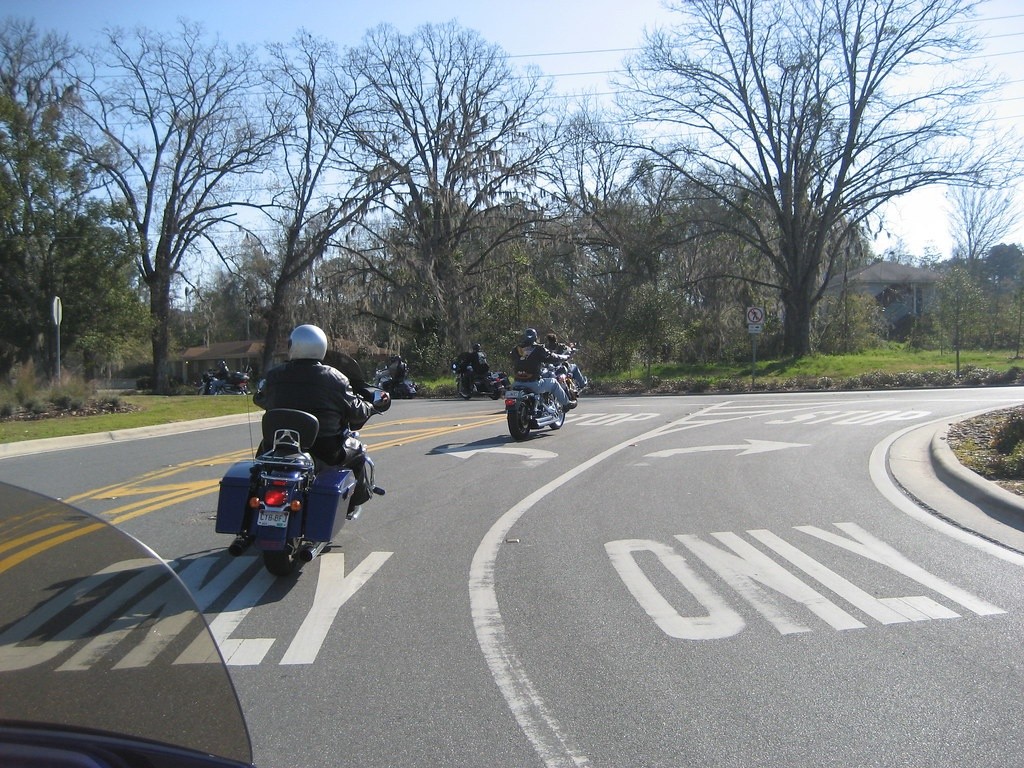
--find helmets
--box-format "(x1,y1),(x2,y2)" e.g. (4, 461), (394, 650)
(218, 360), (225, 366)
(289, 325), (327, 360)
(394, 356), (401, 360)
(473, 343), (481, 350)
(524, 328), (537, 339)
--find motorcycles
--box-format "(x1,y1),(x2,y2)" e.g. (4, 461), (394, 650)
(199, 368), (251, 396)
(503, 364), (578, 444)
(451, 352), (507, 401)
(374, 364), (418, 400)
(215, 348), (387, 580)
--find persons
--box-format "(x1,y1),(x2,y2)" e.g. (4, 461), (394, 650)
(458, 343), (490, 393)
(512, 328), (587, 412)
(210, 360), (230, 395)
(253, 324), (375, 502)
(383, 354), (408, 396)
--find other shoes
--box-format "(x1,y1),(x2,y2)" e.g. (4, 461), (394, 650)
(580, 376), (587, 389)
(351, 485), (372, 505)
(563, 400), (577, 412)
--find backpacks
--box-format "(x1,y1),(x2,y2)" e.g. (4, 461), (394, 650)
(396, 363), (407, 381)
(473, 350), (490, 374)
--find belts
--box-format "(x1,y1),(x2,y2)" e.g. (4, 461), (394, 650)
(514, 378), (537, 383)
